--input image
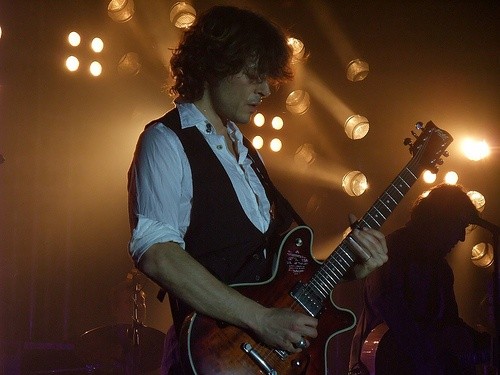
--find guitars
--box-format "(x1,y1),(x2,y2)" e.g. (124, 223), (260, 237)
(361, 324), (500, 375)
(184, 118), (455, 375)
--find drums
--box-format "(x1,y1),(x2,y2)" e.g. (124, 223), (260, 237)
(77, 322), (166, 375)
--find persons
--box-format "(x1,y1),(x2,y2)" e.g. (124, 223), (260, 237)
(347, 184), (481, 375)
(125, 6), (389, 353)
(159, 324), (181, 375)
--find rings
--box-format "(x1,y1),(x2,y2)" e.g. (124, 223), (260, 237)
(295, 338), (306, 349)
